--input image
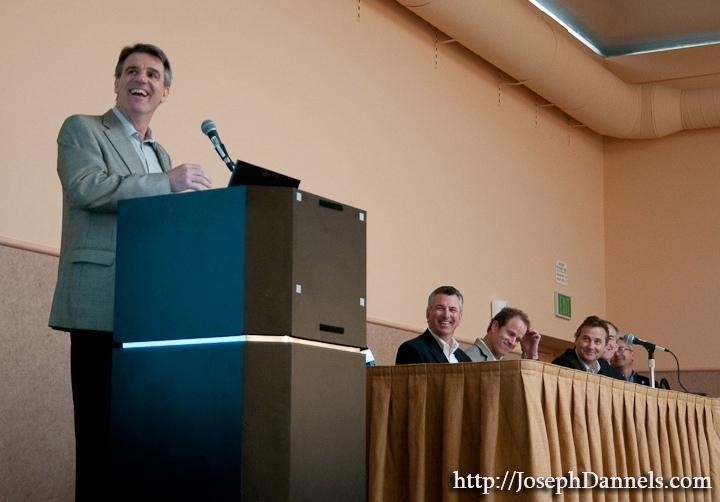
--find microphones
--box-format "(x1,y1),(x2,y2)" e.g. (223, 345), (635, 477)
(624, 333), (669, 353)
(202, 120), (238, 172)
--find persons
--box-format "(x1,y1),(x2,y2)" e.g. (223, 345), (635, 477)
(552, 315), (658, 388)
(49, 41), (210, 501)
(395, 286), (473, 364)
(464, 307), (541, 363)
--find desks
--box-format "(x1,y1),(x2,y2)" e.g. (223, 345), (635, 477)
(365, 359), (719, 502)
